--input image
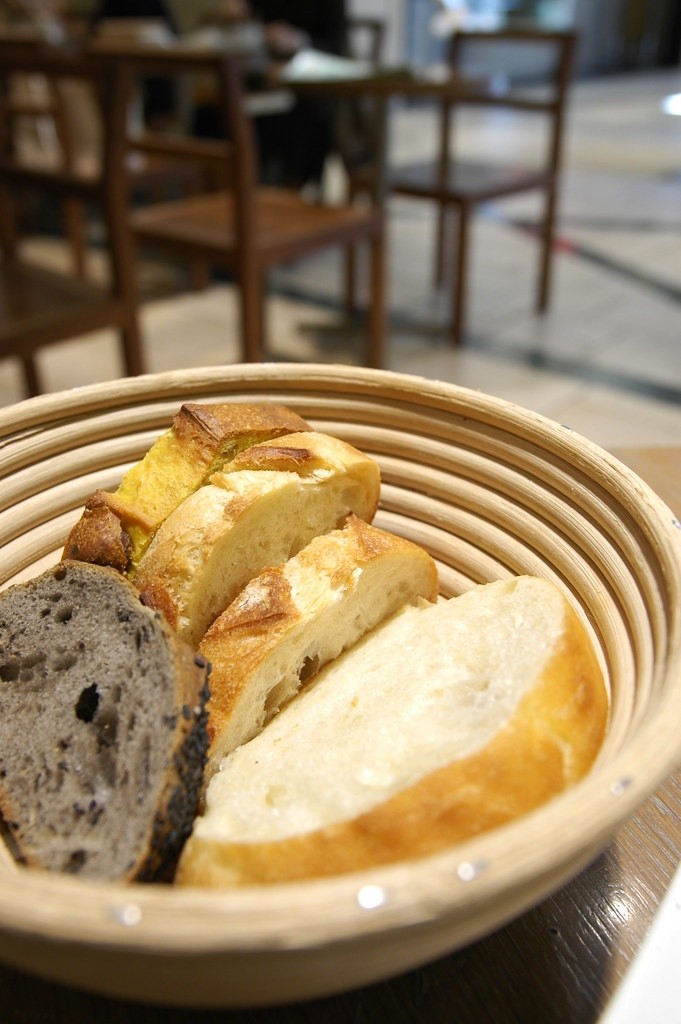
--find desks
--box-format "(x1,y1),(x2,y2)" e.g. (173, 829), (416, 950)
(142, 59), (412, 309)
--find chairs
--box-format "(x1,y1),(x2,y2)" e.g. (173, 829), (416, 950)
(1, 45), (148, 404)
(104, 49), (392, 376)
(391, 31), (574, 344)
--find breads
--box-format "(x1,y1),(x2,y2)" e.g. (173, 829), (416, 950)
(170, 575), (607, 886)
(193, 514), (440, 811)
(0, 558), (219, 888)
(60, 400), (317, 580)
(132, 431), (381, 651)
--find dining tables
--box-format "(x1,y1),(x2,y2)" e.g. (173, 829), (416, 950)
(0, 445), (681, 1024)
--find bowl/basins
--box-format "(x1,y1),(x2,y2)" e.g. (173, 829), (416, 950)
(1, 357), (681, 1003)
